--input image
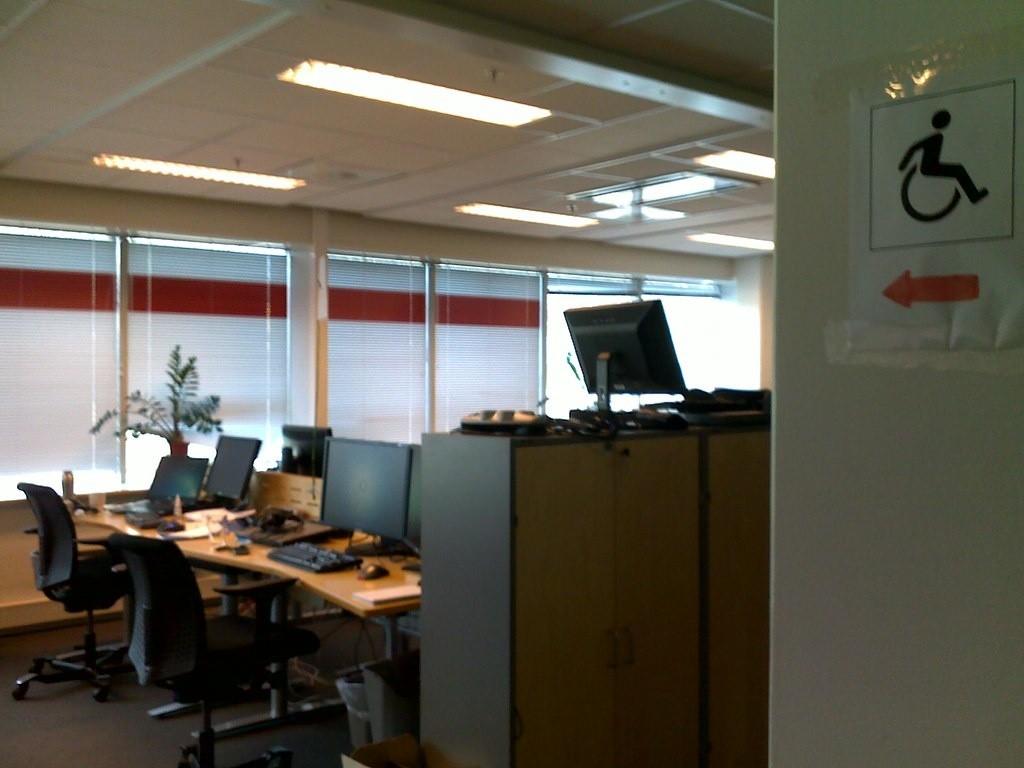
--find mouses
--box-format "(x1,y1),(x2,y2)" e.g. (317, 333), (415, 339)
(358, 563), (389, 579)
(158, 521), (185, 531)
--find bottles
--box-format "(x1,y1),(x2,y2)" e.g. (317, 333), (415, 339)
(62, 471), (73, 500)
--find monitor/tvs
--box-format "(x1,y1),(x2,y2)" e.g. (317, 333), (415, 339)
(204, 436), (262, 510)
(318, 436), (413, 556)
(281, 425), (332, 477)
(565, 299), (687, 421)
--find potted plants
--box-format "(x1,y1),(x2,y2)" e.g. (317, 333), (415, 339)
(90, 343), (222, 456)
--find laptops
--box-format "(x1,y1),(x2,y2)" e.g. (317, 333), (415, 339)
(110, 457), (209, 516)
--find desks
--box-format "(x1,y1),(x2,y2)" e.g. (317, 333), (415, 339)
(73, 513), (421, 745)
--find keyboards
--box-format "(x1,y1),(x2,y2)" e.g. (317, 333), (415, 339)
(126, 512), (165, 529)
(267, 542), (363, 575)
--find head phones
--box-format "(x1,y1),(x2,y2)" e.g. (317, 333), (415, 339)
(257, 507), (305, 534)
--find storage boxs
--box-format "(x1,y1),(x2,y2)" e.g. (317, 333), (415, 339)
(339, 733), (421, 768)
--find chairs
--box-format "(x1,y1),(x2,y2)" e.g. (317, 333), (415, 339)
(11, 482), (136, 703)
(104, 534), (322, 768)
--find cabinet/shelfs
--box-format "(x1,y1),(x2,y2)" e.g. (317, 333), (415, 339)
(421, 406), (770, 768)
